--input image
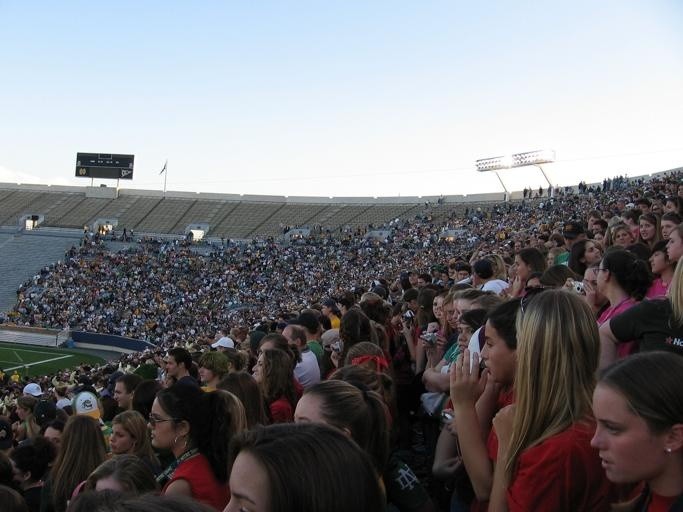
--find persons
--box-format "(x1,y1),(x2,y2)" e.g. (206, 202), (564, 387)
(414, 167), (682, 512)
(1, 220), (412, 512)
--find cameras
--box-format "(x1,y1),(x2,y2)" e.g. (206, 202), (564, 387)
(571, 280), (586, 294)
(420, 332), (441, 346)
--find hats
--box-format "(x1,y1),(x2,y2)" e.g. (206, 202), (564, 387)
(72, 390), (101, 421)
(23, 383), (43, 397)
(211, 337), (236, 350)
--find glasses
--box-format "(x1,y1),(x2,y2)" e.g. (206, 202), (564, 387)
(148, 412), (172, 426)
(593, 268), (610, 275)
